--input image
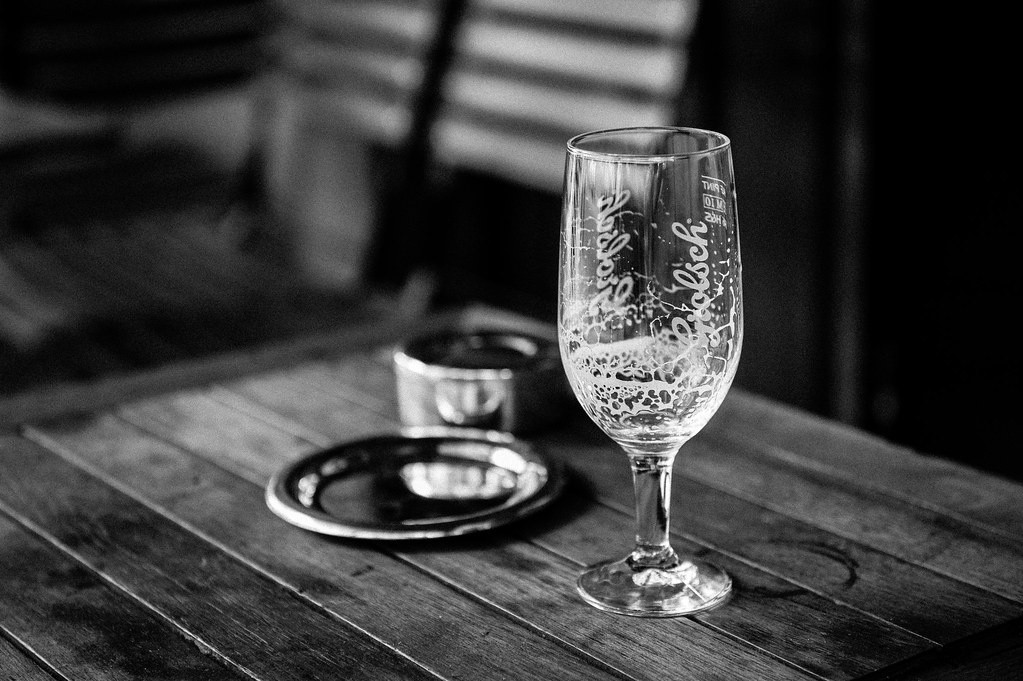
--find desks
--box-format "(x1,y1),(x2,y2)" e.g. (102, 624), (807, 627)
(1, 301), (1023, 680)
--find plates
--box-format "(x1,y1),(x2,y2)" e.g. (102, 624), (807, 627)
(265, 426), (564, 540)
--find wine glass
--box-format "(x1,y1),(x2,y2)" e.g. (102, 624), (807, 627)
(557, 127), (744, 617)
